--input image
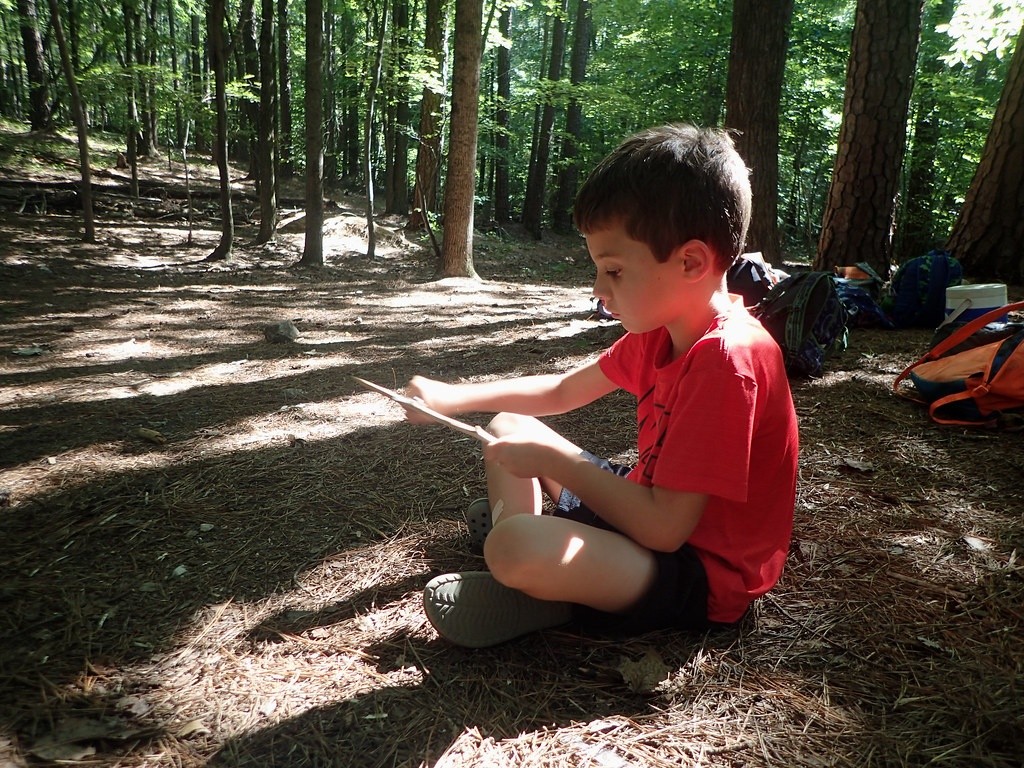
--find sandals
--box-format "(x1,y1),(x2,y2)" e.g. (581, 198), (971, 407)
(466, 498), (493, 548)
(422, 571), (575, 650)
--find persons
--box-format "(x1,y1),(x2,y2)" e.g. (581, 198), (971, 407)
(402, 125), (800, 649)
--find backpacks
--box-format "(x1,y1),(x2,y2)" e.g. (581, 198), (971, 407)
(815, 262), (890, 330)
(752, 270), (851, 381)
(892, 247), (963, 327)
(892, 300), (1024, 426)
(727, 252), (776, 307)
